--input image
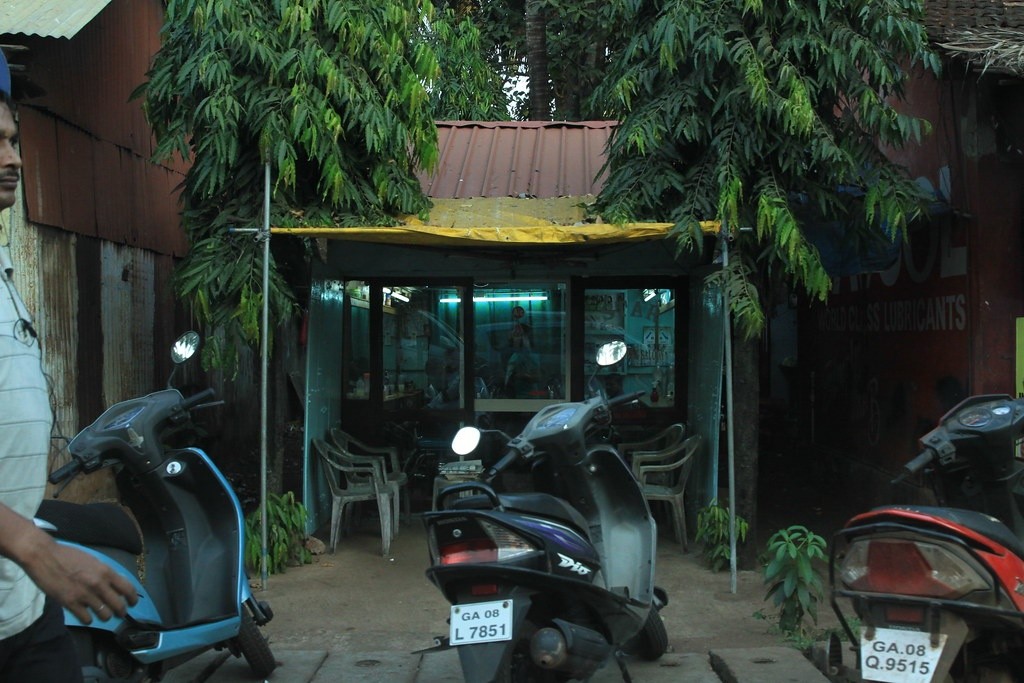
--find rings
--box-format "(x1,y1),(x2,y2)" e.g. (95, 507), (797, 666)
(95, 603), (106, 613)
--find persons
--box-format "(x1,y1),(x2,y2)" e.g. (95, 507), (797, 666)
(498, 304), (538, 399)
(0, 87), (139, 683)
(340, 363), (434, 497)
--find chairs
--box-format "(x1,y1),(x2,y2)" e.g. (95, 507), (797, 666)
(329, 427), (410, 536)
(631, 432), (703, 554)
(617, 422), (687, 527)
(313, 438), (392, 560)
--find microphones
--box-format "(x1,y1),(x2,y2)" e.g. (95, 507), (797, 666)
(22, 318), (37, 338)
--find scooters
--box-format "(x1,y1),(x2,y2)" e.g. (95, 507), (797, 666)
(828, 389), (1024, 683)
(418, 338), (668, 682)
(35, 333), (275, 683)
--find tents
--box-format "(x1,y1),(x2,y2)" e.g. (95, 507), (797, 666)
(245, 194), (745, 593)
